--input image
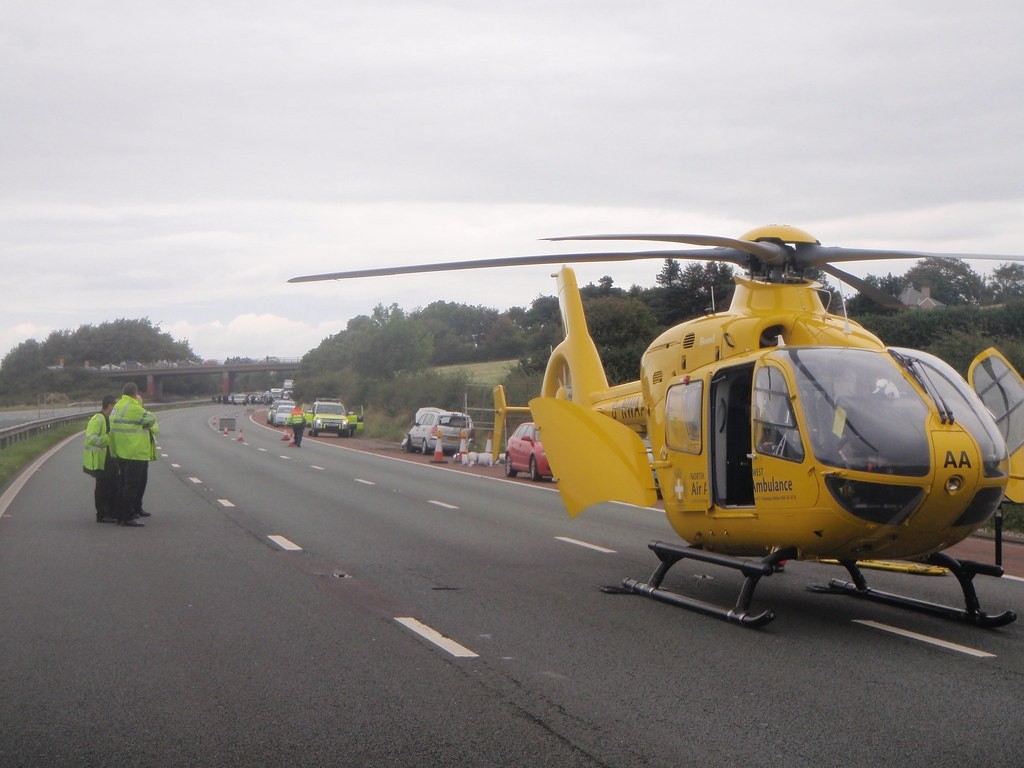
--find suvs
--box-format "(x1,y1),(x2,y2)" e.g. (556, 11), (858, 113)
(122, 362), (148, 369)
(302, 397), (365, 439)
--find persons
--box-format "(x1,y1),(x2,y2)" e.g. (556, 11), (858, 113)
(82, 382), (160, 527)
(212, 393), (234, 404)
(245, 394), (273, 405)
(285, 404), (307, 448)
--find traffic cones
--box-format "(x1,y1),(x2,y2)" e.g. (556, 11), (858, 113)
(223, 425), (230, 435)
(484, 430), (493, 454)
(430, 429), (447, 463)
(236, 427), (244, 442)
(282, 417), (291, 441)
(454, 431), (468, 464)
(213, 416), (217, 429)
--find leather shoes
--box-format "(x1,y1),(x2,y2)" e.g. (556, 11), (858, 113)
(97, 514), (118, 522)
(121, 519), (144, 526)
(134, 514), (140, 518)
(139, 508), (151, 516)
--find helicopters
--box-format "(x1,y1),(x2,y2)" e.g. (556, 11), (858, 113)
(291, 223), (1023, 630)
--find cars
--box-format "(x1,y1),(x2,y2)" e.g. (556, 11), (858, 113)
(101, 364), (120, 370)
(227, 379), (297, 428)
(408, 411), (475, 454)
(504, 423), (555, 481)
